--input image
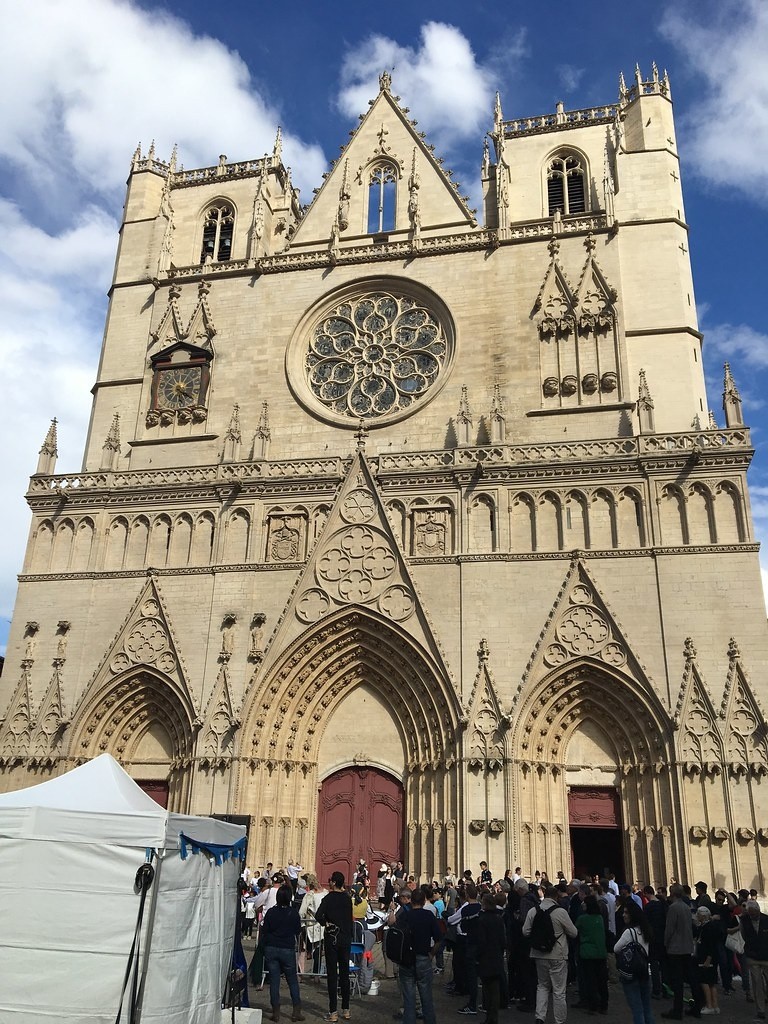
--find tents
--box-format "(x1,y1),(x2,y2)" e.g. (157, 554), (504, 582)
(0, 754), (246, 1024)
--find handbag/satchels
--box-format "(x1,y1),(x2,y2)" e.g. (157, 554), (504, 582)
(725, 914), (745, 954)
(616, 926), (649, 984)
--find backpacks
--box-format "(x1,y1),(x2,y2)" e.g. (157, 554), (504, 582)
(528, 903), (564, 953)
(385, 926), (416, 968)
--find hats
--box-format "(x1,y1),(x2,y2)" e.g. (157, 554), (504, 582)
(380, 864), (388, 872)
(433, 888), (444, 895)
(619, 884), (631, 893)
(694, 881), (707, 889)
(391, 862), (397, 869)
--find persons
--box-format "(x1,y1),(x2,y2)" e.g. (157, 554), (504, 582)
(259, 885), (305, 1022)
(613, 902), (654, 1024)
(352, 858), (416, 915)
(315, 872), (353, 1021)
(522, 887), (578, 1024)
(351, 882), (368, 965)
(741, 901), (768, 1024)
(389, 889), (443, 1024)
(477, 894), (506, 1024)
(660, 884), (701, 1019)
(241, 859), (330, 984)
(420, 861), (768, 1014)
(379, 71), (391, 91)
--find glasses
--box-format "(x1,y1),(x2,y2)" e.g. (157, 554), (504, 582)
(328, 878), (331, 883)
(717, 897), (725, 902)
(435, 892), (438, 894)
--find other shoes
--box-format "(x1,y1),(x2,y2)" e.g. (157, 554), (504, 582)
(242, 935), (251, 940)
(652, 975), (766, 1019)
(432, 967), (444, 975)
(416, 1012), (424, 1019)
(393, 1013), (403, 1020)
(443, 980), (608, 1024)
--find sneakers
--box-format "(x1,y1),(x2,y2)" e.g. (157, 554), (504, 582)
(341, 1008), (351, 1019)
(323, 1011), (339, 1022)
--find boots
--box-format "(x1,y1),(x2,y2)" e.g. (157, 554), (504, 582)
(269, 1006), (280, 1022)
(292, 1004), (305, 1022)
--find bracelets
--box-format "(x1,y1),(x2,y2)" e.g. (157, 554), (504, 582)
(389, 909), (394, 912)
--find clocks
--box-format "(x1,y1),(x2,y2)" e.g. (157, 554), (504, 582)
(153, 364), (202, 408)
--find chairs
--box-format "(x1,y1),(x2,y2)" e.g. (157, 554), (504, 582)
(335, 941), (364, 1000)
